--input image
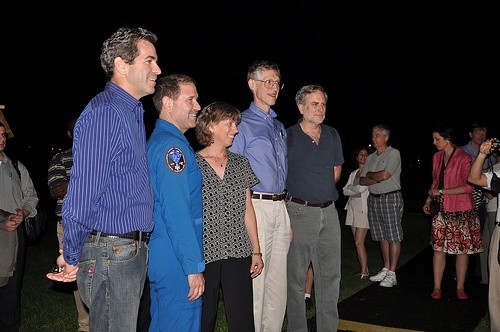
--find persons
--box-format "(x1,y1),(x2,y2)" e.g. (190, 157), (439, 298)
(283, 85), (341, 332)
(0, 122), (40, 332)
(146, 73), (206, 332)
(46, 28), (162, 332)
(360, 126), (405, 286)
(227, 63), (293, 332)
(459, 124), (500, 332)
(191, 102), (263, 332)
(424, 129), (484, 299)
(342, 147), (370, 279)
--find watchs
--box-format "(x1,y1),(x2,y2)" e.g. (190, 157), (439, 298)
(439, 188), (444, 195)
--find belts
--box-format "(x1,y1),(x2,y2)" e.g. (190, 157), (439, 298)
(250, 194), (286, 201)
(287, 195), (334, 208)
(90, 229), (151, 245)
(369, 189), (401, 198)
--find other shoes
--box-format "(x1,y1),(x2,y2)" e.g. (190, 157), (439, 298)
(359, 266), (369, 279)
(456, 290), (467, 299)
(305, 297), (313, 311)
(431, 288), (441, 298)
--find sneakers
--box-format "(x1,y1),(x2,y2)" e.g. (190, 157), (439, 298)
(379, 270), (397, 287)
(369, 267), (389, 281)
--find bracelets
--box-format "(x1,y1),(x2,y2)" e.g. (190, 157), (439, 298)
(251, 252), (262, 257)
(479, 152), (486, 158)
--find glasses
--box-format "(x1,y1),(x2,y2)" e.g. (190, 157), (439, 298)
(255, 79), (285, 90)
(473, 130), (486, 136)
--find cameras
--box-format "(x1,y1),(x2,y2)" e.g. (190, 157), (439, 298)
(491, 139), (500, 149)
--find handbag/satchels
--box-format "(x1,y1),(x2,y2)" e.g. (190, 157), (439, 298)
(429, 197), (441, 216)
(490, 172), (500, 194)
